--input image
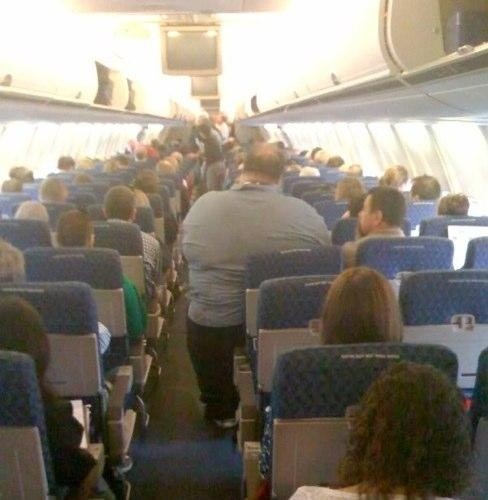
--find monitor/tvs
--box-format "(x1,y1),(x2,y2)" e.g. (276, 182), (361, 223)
(160, 25), (221, 75)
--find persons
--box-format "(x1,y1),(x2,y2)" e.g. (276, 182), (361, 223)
(1, 180), (25, 191)
(100, 185), (163, 299)
(54, 209), (150, 340)
(334, 175), (365, 201)
(57, 124), (176, 184)
(176, 142), (340, 434)
(341, 192), (370, 222)
(128, 185), (161, 209)
(340, 187), (406, 271)
(1, 240), (116, 420)
(39, 179), (69, 210)
(378, 166), (414, 194)
(408, 174), (442, 206)
(276, 142), (340, 178)
(176, 142), (247, 192)
(176, 110), (272, 142)
(258, 266), (405, 478)
(434, 193), (470, 216)
(10, 167), (35, 183)
(14, 200), (51, 227)
(2, 295), (97, 489)
(340, 158), (363, 175)
(283, 359), (477, 499)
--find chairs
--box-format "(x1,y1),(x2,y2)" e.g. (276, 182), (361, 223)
(169, 221), (179, 246)
(23, 245), (153, 394)
(413, 220), (419, 230)
(234, 273), (340, 453)
(0, 350), (106, 499)
(332, 219), (413, 246)
(419, 215), (488, 268)
(325, 220), (331, 231)
(133, 221), (168, 243)
(242, 342), (459, 500)
(233, 244), (343, 387)
(461, 236), (488, 269)
(225, 146), (439, 220)
(354, 234), (454, 301)
(398, 268), (488, 412)
(0, 279), (136, 457)
(92, 221), (169, 343)
(0, 147), (202, 221)
(332, 220), (336, 228)
(0, 219), (52, 251)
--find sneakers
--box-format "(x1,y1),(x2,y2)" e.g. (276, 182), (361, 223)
(111, 455), (133, 475)
(205, 406), (240, 428)
